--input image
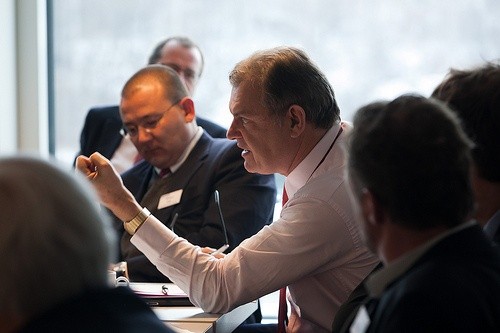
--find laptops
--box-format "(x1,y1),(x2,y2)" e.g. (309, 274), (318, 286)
(117, 282), (195, 307)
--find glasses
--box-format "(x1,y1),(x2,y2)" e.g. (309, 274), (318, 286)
(120, 99), (180, 137)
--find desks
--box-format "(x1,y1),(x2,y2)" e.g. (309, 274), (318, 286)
(152, 298), (258, 333)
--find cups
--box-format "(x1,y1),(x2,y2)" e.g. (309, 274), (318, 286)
(106, 270), (129, 288)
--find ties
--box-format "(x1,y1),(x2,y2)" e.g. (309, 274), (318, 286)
(278, 182), (289, 333)
(158, 168), (170, 177)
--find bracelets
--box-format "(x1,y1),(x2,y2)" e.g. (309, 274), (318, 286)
(116, 262), (127, 282)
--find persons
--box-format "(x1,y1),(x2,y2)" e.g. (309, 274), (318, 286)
(102, 64), (277, 324)
(429, 62), (500, 246)
(344, 94), (500, 333)
(74, 36), (229, 176)
(75, 46), (381, 333)
(0, 154), (177, 333)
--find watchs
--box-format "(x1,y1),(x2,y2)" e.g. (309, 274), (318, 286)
(123, 207), (150, 235)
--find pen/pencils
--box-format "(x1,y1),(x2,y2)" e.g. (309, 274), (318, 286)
(210, 244), (230, 258)
